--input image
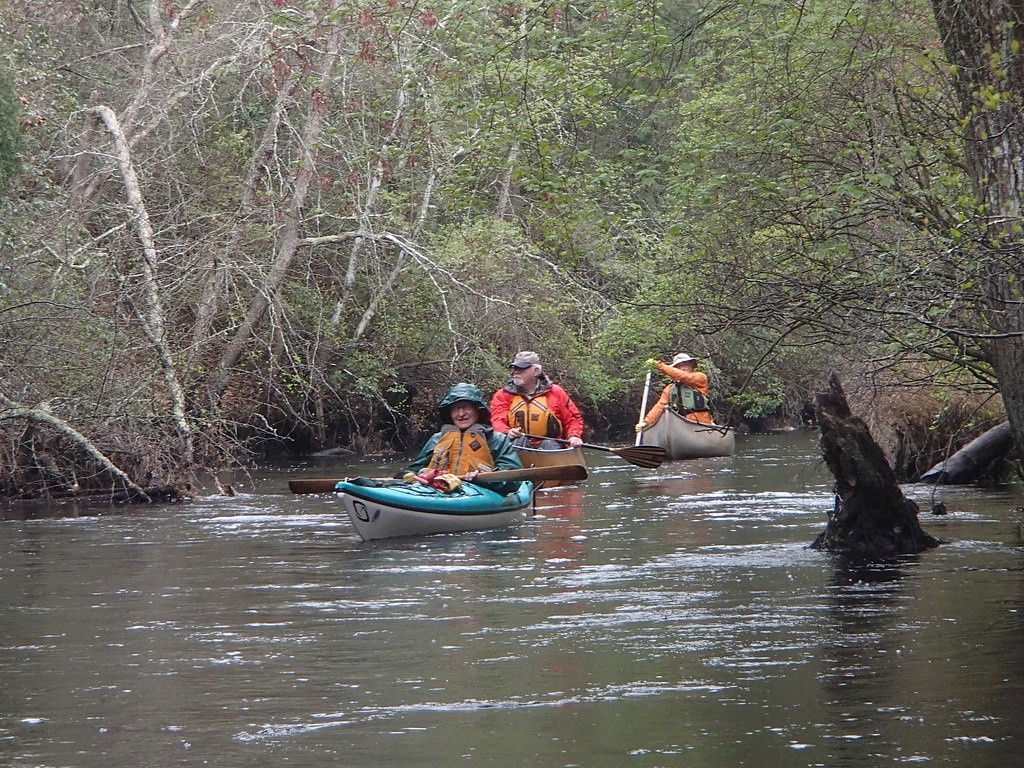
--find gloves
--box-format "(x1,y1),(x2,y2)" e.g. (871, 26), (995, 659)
(643, 358), (657, 370)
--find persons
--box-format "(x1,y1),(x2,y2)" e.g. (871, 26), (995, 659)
(489, 352), (585, 450)
(635, 352), (712, 432)
(399, 382), (524, 496)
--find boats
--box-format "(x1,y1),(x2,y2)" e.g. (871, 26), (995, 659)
(639, 403), (735, 463)
(333, 475), (536, 542)
(506, 442), (590, 488)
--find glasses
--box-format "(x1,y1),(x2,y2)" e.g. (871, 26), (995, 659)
(450, 405), (474, 412)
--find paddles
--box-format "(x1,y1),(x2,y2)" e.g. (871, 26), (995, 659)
(287, 462), (589, 496)
(517, 431), (667, 471)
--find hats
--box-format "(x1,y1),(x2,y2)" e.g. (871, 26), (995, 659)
(668, 353), (699, 369)
(508, 351), (540, 369)
(437, 383), (492, 417)
